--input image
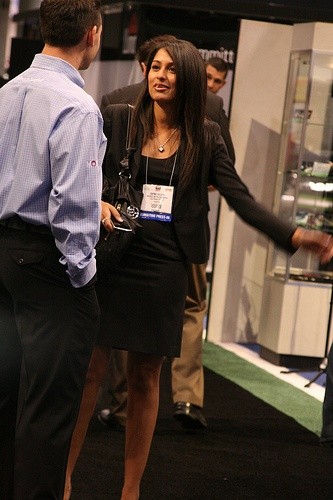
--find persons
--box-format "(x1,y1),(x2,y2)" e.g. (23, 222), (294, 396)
(203, 56), (228, 94)
(62, 38), (332, 500)
(0, 0), (110, 500)
(95, 33), (236, 437)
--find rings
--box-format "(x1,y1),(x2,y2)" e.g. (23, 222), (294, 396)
(101, 217), (107, 225)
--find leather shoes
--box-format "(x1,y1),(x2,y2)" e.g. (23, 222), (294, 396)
(173, 402), (208, 438)
(98, 408), (128, 433)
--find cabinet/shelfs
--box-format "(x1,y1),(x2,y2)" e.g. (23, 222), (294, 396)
(255, 49), (333, 370)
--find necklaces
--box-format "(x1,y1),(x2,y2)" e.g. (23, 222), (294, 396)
(151, 126), (180, 154)
